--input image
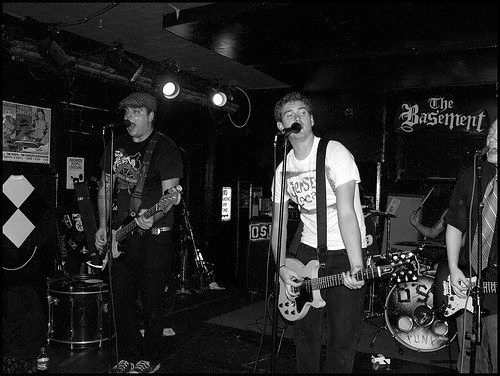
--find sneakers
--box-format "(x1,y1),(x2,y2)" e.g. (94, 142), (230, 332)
(113, 359), (131, 373)
(127, 358), (161, 373)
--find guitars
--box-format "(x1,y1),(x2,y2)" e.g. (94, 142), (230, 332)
(101, 184), (183, 272)
(273, 251), (422, 321)
(432, 260), (483, 321)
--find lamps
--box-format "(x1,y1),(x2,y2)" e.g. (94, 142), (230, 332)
(104, 44), (144, 82)
(47, 38), (77, 70)
(152, 64), (182, 100)
(206, 83), (234, 107)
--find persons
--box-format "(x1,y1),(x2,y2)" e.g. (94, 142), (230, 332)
(95, 92), (184, 374)
(443, 119), (498, 374)
(271, 92), (368, 376)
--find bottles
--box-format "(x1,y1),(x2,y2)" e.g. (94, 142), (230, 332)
(36, 346), (49, 375)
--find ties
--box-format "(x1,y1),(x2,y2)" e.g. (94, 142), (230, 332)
(472, 175), (497, 274)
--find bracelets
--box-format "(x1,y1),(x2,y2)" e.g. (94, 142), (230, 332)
(280, 265), (286, 268)
(352, 266), (361, 271)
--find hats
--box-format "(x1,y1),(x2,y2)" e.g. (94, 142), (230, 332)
(119, 93), (157, 111)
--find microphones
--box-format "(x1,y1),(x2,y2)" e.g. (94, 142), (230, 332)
(276, 122), (301, 136)
(104, 119), (131, 129)
(476, 146), (490, 155)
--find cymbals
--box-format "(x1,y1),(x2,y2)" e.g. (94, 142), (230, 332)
(368, 209), (396, 218)
(396, 240), (447, 247)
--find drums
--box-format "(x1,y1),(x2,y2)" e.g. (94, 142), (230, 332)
(373, 251), (457, 352)
(45, 276), (116, 351)
